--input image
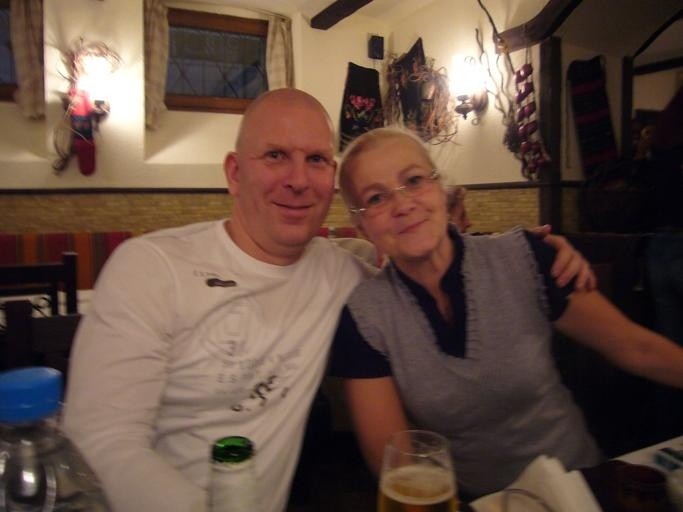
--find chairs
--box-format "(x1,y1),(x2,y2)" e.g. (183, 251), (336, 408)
(0, 252), (77, 367)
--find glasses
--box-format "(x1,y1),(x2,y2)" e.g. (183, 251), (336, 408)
(349, 169), (440, 216)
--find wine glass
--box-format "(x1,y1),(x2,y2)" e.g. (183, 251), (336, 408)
(376, 428), (457, 512)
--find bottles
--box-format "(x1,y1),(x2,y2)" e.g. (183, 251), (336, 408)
(0, 366), (111, 512)
(208, 435), (258, 512)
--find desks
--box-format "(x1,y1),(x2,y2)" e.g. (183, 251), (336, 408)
(0, 290), (90, 320)
(579, 437), (683, 512)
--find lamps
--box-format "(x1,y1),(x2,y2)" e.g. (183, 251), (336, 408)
(446, 54), (490, 118)
(47, 39), (119, 128)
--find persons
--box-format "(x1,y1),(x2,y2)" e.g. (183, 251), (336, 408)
(641, 83), (683, 348)
(326, 124), (683, 512)
(60, 87), (604, 512)
